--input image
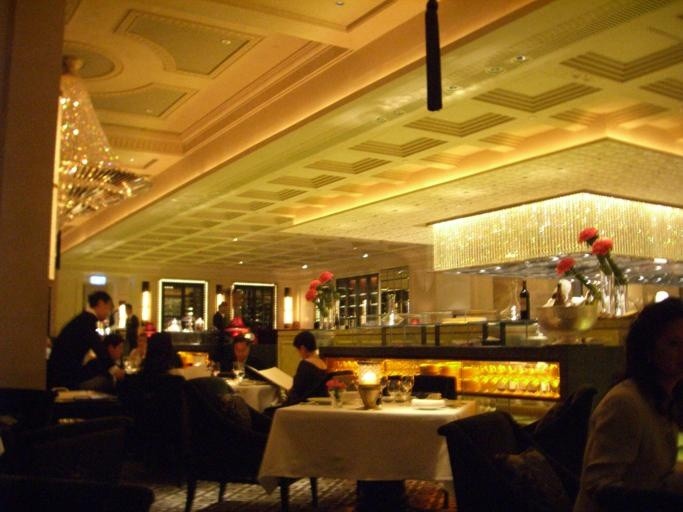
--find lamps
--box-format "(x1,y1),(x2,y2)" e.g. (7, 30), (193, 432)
(216, 285), (223, 311)
(141, 281), (152, 321)
(58, 55), (154, 233)
(119, 301), (126, 328)
(283, 287), (293, 329)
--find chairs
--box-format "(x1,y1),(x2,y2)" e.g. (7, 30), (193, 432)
(413, 374), (596, 512)
(0, 357), (352, 512)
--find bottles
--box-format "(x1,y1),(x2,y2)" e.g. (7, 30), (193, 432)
(519, 280), (530, 320)
(554, 283), (566, 306)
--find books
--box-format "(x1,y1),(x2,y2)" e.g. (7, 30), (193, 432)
(244, 364), (294, 391)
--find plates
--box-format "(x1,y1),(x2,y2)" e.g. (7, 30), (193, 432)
(412, 405), (444, 409)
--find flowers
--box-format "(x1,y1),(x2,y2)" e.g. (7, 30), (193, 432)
(306, 271), (339, 316)
(558, 228), (625, 300)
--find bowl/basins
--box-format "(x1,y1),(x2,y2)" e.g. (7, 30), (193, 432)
(536, 305), (598, 332)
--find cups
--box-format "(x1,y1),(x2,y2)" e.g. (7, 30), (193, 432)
(330, 390), (344, 408)
(358, 384), (383, 409)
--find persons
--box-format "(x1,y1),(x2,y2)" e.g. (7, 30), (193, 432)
(262, 330), (327, 417)
(571, 295), (683, 512)
(45, 289), (266, 419)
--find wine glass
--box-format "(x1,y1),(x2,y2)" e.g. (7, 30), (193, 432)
(387, 377), (401, 409)
(401, 375), (414, 392)
